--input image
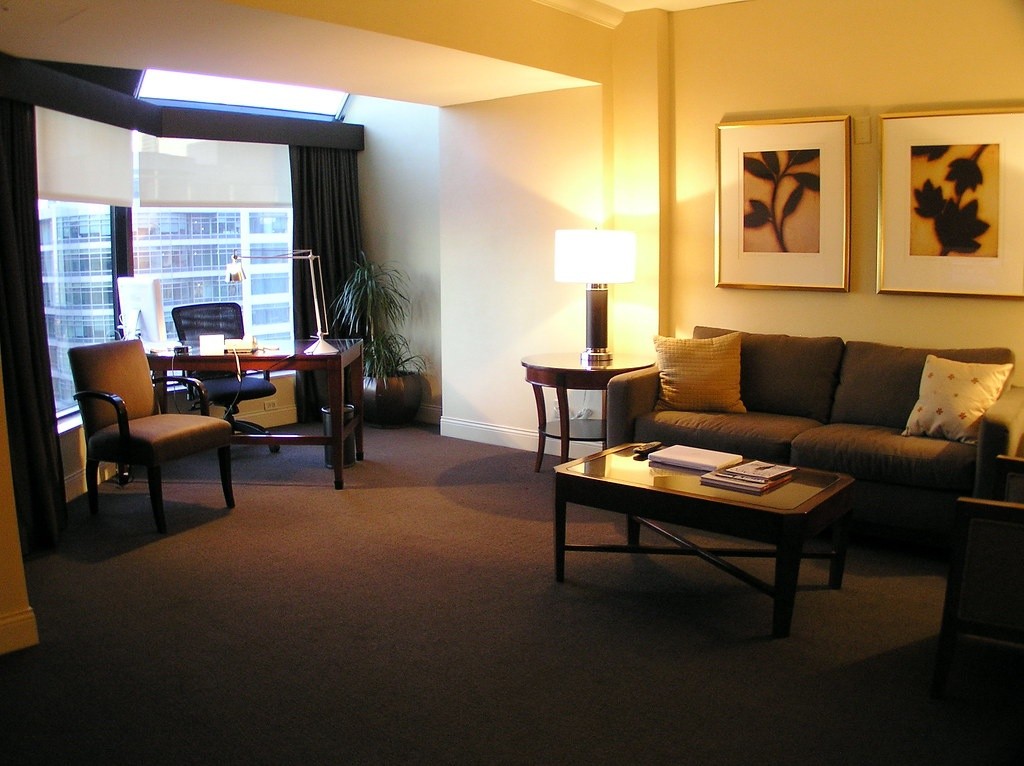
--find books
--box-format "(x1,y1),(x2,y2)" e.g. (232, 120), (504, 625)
(699, 458), (798, 497)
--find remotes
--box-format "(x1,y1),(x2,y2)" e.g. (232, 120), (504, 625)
(633, 441), (661, 454)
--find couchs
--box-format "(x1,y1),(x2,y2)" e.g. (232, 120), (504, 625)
(923, 454), (1024, 671)
(605, 327), (1024, 557)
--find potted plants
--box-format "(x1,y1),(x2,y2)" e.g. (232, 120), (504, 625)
(331, 251), (424, 429)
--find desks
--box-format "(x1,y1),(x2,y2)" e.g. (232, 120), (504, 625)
(553, 443), (858, 638)
(521, 352), (656, 471)
(142, 337), (365, 489)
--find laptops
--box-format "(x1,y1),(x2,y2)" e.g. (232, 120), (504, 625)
(648, 445), (743, 471)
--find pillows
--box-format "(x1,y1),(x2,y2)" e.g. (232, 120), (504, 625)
(654, 331), (747, 414)
(899, 355), (1013, 443)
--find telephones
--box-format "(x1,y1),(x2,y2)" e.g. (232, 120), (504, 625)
(225, 334), (256, 353)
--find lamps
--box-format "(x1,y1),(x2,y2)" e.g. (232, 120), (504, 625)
(225, 248), (338, 355)
(554, 229), (637, 360)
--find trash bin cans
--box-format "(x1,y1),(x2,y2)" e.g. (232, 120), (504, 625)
(321, 404), (356, 469)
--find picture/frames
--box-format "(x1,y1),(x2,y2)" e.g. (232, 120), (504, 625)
(715, 114), (851, 292)
(875, 109), (1024, 298)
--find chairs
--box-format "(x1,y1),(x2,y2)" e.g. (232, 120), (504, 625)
(171, 302), (282, 452)
(70, 340), (235, 534)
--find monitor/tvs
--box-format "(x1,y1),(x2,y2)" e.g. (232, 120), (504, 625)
(117, 277), (168, 354)
